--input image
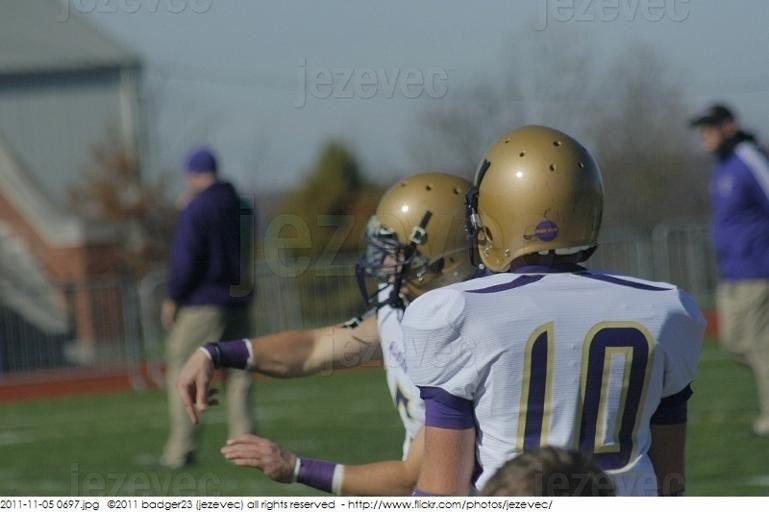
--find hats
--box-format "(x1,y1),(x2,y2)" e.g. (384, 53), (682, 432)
(689, 105), (732, 125)
(186, 150), (217, 172)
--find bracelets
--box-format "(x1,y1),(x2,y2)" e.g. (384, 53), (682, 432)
(199, 337), (254, 373)
(292, 456), (344, 495)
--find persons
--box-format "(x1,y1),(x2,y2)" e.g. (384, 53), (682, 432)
(481, 445), (617, 496)
(175, 173), (492, 495)
(399, 125), (706, 495)
(155, 145), (258, 472)
(688, 103), (768, 438)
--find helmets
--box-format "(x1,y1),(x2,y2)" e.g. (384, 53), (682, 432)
(367, 125), (604, 296)
